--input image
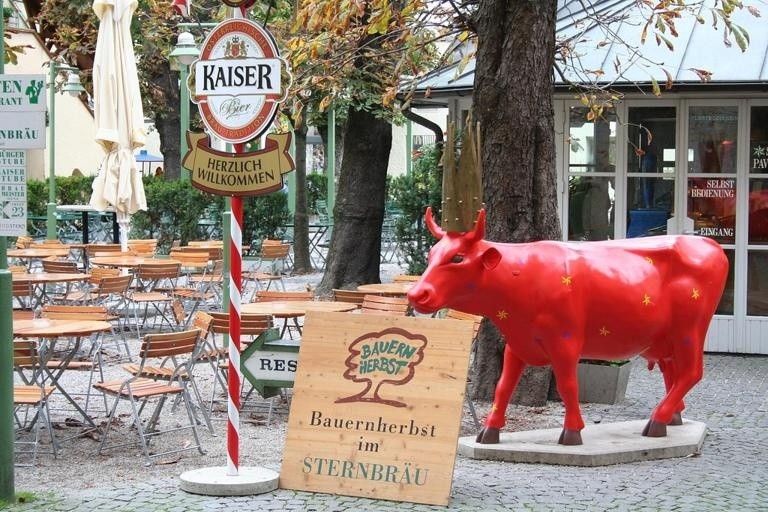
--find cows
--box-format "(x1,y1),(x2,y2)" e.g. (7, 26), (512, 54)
(406, 206), (730, 448)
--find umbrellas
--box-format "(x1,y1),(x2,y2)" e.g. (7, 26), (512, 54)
(87, 0), (150, 275)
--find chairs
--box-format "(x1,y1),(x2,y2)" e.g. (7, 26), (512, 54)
(6, 199), (486, 470)
